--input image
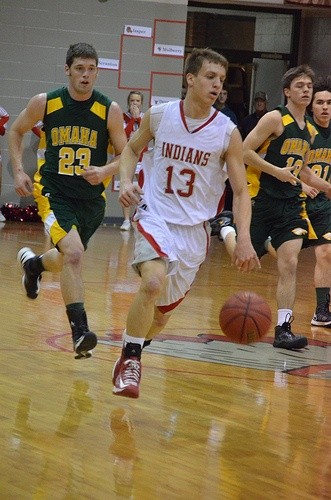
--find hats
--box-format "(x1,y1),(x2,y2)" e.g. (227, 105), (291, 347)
(254, 91), (267, 102)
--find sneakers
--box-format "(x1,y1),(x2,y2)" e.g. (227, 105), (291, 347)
(17, 246), (42, 299)
(273, 313), (307, 348)
(209, 211), (234, 241)
(72, 325), (97, 359)
(120, 221), (131, 231)
(112, 343), (142, 399)
(311, 307), (331, 326)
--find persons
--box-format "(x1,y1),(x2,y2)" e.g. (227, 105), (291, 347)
(241, 90), (271, 154)
(210, 62), (331, 350)
(213, 85), (239, 127)
(258, 75), (331, 329)
(111, 45), (262, 399)
(9, 41), (128, 359)
(120, 91), (153, 232)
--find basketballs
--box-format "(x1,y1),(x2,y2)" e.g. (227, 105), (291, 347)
(219, 291), (273, 343)
